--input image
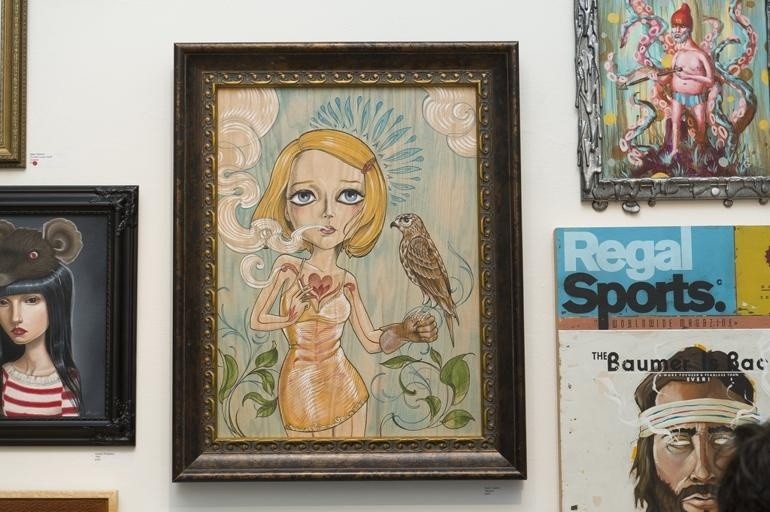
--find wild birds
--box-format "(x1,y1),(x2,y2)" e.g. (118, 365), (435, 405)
(388, 212), (461, 348)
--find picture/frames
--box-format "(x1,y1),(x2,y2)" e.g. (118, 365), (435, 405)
(1, 491), (120, 511)
(1, 1), (28, 171)
(573, 1), (770, 215)
(174, 40), (528, 481)
(0, 185), (137, 449)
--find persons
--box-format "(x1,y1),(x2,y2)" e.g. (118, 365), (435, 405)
(247, 127), (438, 437)
(716, 420), (770, 511)
(0, 228), (87, 417)
(649, 3), (716, 172)
(629, 347), (758, 512)
